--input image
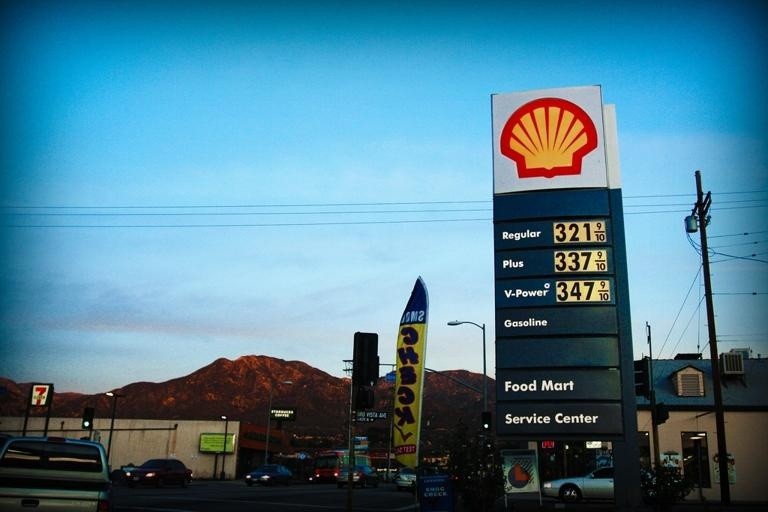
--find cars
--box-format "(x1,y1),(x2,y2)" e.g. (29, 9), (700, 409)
(395, 469), (416, 490)
(245, 464), (291, 488)
(338, 465), (384, 489)
(126, 459), (192, 487)
(543, 466), (651, 500)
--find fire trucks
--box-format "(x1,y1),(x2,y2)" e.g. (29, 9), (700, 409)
(314, 450), (372, 482)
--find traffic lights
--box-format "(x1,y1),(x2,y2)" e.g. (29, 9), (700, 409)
(481, 412), (492, 431)
(82, 407), (94, 430)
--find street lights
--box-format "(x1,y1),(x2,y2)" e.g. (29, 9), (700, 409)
(220, 415), (227, 479)
(269, 408), (297, 428)
(264, 381), (293, 466)
(106, 391), (124, 457)
(446, 321), (488, 414)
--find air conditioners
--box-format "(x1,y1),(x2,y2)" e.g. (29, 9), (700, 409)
(720, 352), (745, 380)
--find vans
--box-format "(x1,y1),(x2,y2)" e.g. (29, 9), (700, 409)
(1, 437), (112, 511)
